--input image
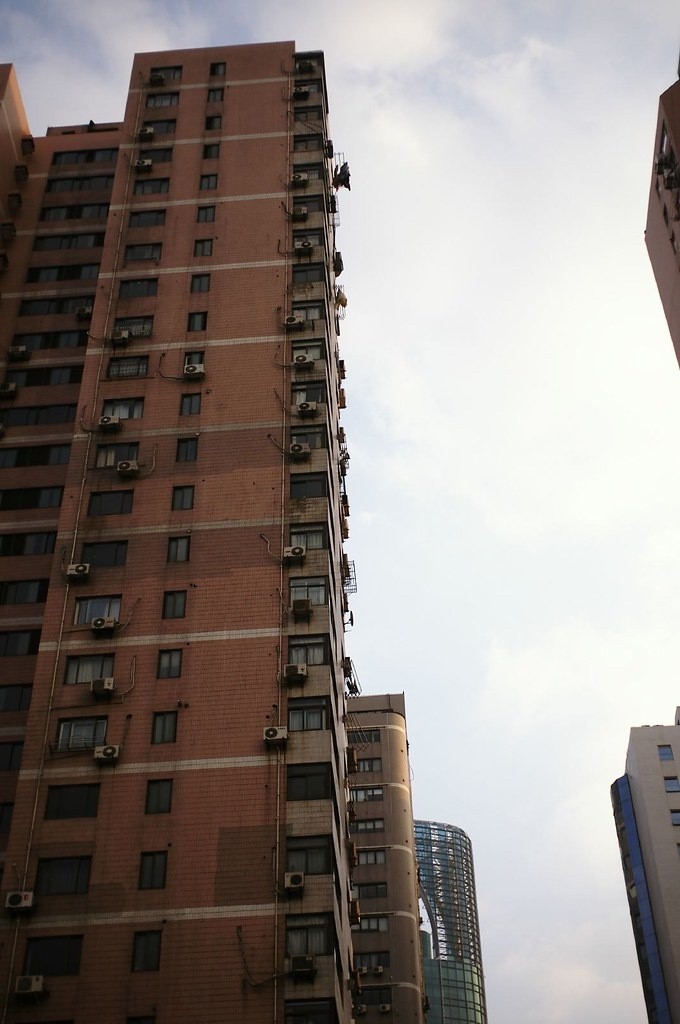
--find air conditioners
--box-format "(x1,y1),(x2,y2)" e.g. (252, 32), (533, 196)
(76, 306), (93, 315)
(116, 459), (139, 476)
(94, 744), (119, 760)
(89, 677), (117, 694)
(91, 616), (117, 631)
(13, 974), (49, 1000)
(66, 564), (91, 579)
(348, 748), (392, 1016)
(98, 415), (120, 431)
(0, 381), (17, 393)
(135, 158), (153, 167)
(138, 127), (155, 135)
(259, 64), (352, 745)
(284, 872), (307, 895)
(289, 954), (319, 978)
(5, 890), (40, 914)
(184, 363), (205, 377)
(10, 344), (26, 358)
(109, 329), (129, 342)
